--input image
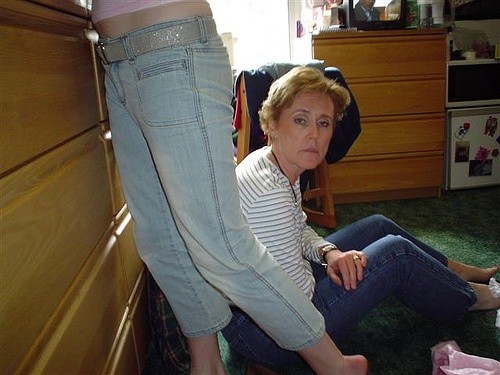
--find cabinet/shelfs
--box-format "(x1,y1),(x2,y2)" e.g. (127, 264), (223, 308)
(0, 0), (143, 375)
(309, 27), (446, 201)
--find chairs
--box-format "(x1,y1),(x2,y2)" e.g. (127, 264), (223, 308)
(233, 75), (334, 227)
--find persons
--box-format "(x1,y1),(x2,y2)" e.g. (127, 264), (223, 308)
(353, 0), (380, 21)
(92, 0), (367, 375)
(219, 67), (500, 369)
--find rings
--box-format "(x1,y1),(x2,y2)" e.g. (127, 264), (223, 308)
(352, 255), (359, 261)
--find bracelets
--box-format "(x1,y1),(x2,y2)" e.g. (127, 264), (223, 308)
(317, 244), (338, 268)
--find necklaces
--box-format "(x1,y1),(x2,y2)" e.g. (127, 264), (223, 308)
(270, 149), (298, 207)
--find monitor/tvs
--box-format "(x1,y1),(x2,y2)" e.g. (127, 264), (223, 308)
(343, 0), (407, 31)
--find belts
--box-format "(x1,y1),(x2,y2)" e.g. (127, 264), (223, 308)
(86, 17), (218, 63)
